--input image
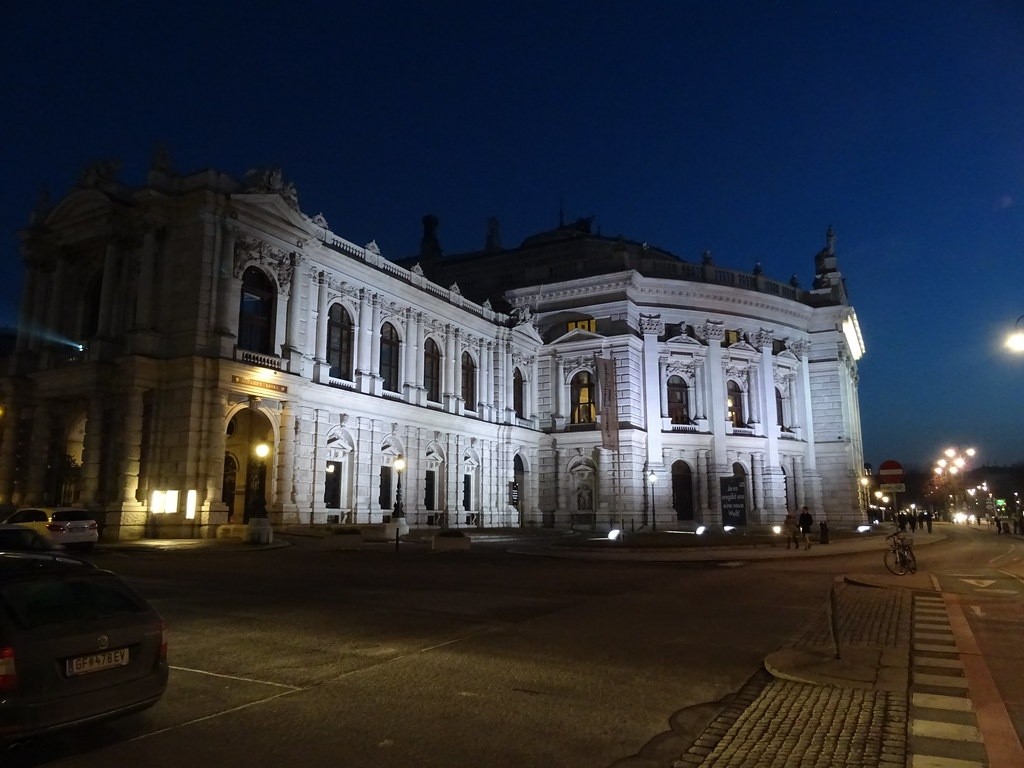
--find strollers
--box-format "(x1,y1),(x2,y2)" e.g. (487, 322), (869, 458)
(1002, 523), (1011, 535)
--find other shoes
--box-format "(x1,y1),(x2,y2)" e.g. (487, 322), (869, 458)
(786, 547), (790, 549)
(804, 548), (807, 550)
(808, 544), (811, 549)
(796, 545), (798, 549)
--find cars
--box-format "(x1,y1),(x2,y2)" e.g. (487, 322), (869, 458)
(0, 551), (168, 749)
(0, 506), (99, 551)
(0, 524), (53, 554)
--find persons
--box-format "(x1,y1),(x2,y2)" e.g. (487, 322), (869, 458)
(994, 516), (1002, 534)
(783, 514), (801, 549)
(977, 515), (981, 526)
(898, 509), (933, 533)
(1011, 513), (1019, 534)
(799, 506), (813, 550)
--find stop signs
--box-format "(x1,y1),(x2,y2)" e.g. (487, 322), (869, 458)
(877, 460), (904, 484)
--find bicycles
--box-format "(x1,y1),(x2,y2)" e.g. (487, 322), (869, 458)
(885, 531), (918, 576)
